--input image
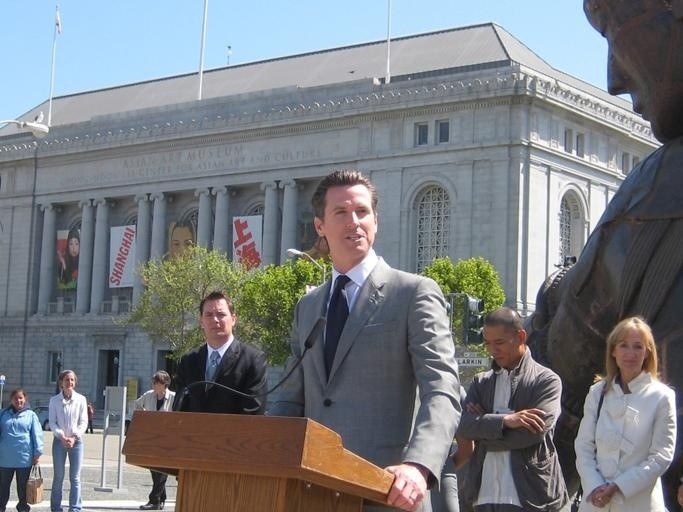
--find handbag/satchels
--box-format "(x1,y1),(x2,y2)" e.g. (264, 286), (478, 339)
(26, 477), (43, 503)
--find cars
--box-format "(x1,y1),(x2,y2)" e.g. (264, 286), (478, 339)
(30, 405), (51, 431)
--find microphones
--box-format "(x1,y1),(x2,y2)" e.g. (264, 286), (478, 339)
(173, 317), (326, 409)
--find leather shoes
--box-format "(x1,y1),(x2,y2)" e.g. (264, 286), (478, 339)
(141, 500), (164, 509)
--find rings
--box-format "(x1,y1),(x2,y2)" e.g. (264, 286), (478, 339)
(410, 493), (416, 502)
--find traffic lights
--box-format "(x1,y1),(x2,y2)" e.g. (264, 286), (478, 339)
(445, 296), (452, 332)
(564, 255), (576, 267)
(462, 296), (483, 344)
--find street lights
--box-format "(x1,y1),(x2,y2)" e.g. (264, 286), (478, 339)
(0, 374), (5, 409)
(0, 119), (48, 140)
(286, 248), (325, 284)
(54, 353), (62, 395)
(111, 356), (120, 386)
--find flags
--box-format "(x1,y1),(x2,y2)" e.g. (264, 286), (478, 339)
(56, 11), (62, 35)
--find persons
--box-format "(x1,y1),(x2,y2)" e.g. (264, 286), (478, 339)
(136, 370), (177, 510)
(173, 292), (267, 414)
(171, 220), (195, 263)
(58, 229), (80, 283)
(459, 307), (571, 512)
(266, 170), (460, 512)
(523, 0), (683, 512)
(49, 370), (88, 512)
(86, 403), (94, 434)
(573, 317), (677, 512)
(0, 388), (44, 512)
(425, 382), (473, 512)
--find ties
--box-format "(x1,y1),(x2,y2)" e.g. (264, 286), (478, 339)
(205, 351), (219, 388)
(326, 275), (350, 379)
